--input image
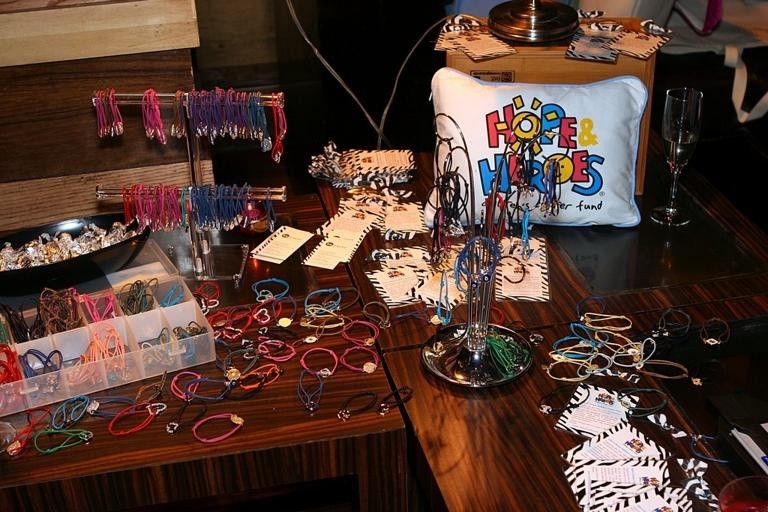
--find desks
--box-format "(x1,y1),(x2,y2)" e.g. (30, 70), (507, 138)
(0, 193), (405, 512)
(320, 154), (768, 512)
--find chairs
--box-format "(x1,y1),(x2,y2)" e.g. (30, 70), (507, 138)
(0, 161), (216, 230)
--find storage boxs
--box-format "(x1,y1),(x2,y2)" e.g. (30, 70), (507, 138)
(443, 17), (657, 198)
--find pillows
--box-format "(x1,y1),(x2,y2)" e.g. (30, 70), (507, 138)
(423, 67), (650, 231)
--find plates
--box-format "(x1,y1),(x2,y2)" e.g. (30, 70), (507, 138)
(0, 211), (152, 295)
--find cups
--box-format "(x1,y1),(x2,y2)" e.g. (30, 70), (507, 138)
(717, 474), (767, 511)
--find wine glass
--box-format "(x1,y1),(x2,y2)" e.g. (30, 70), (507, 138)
(649, 87), (703, 225)
